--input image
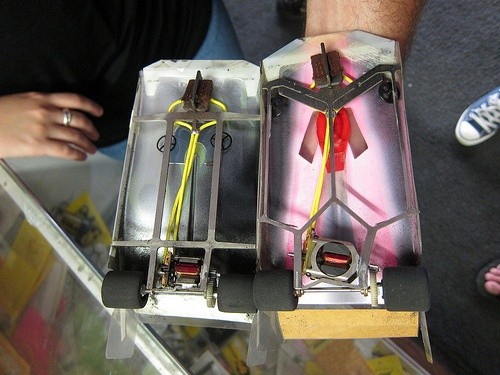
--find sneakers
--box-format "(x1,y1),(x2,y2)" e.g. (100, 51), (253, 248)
(455, 86), (500, 146)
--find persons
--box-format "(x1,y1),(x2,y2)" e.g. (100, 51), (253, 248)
(0, 0), (245, 172)
(475, 259), (500, 302)
(455, 86), (500, 148)
(305, 0), (428, 78)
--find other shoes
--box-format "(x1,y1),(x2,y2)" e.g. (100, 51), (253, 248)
(475, 257), (500, 301)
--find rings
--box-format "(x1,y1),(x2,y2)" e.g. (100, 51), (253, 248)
(62, 108), (71, 126)
